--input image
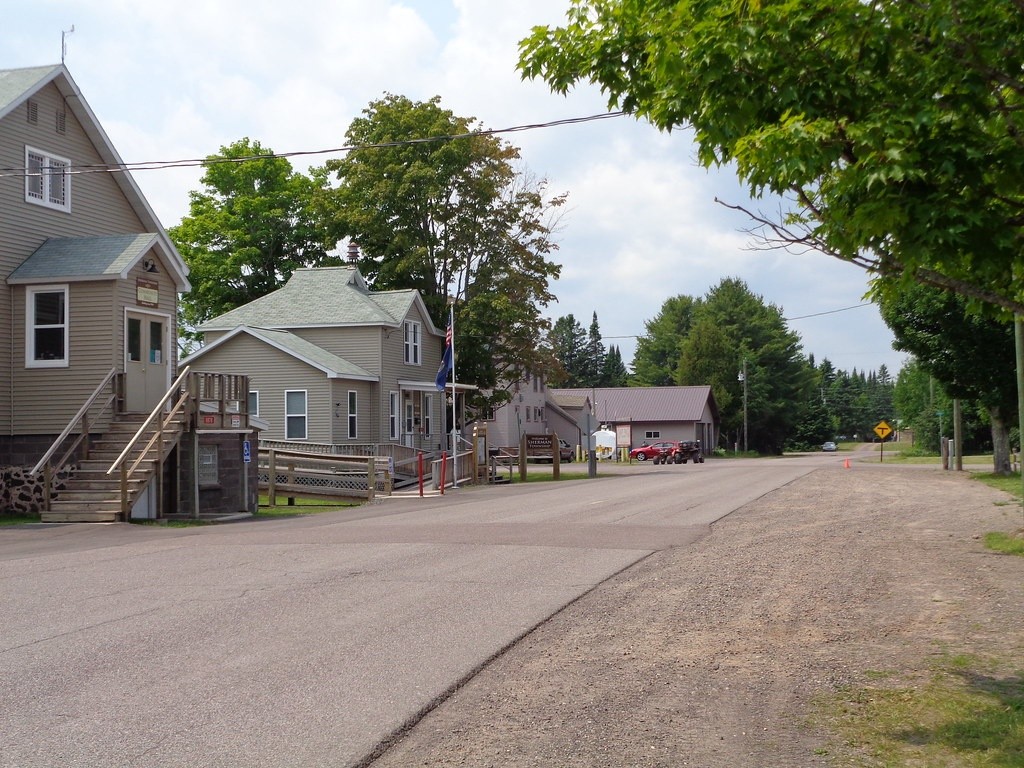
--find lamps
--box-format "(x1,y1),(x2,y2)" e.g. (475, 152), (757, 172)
(587, 401), (598, 405)
(336, 403), (342, 417)
(144, 258), (159, 273)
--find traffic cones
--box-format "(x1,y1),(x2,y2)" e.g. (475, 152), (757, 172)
(844, 458), (851, 468)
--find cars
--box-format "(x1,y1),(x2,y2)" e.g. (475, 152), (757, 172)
(821, 441), (837, 451)
(630, 440), (680, 462)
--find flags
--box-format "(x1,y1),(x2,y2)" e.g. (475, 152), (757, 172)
(436, 314), (452, 393)
